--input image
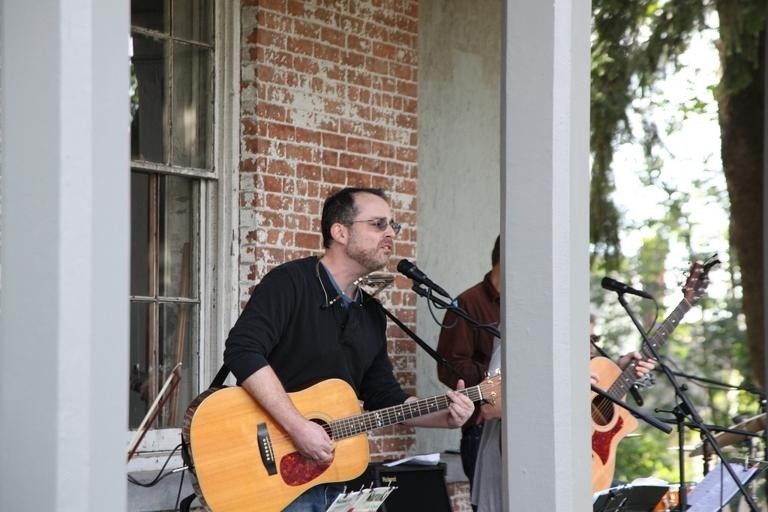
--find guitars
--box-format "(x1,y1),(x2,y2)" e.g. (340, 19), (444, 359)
(590, 257), (722, 494)
(181, 368), (501, 512)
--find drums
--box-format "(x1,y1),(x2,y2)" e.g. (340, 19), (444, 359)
(668, 483), (696, 506)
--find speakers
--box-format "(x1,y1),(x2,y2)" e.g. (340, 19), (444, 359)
(345, 460), (454, 512)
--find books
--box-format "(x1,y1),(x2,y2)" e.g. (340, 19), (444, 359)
(675, 460), (758, 511)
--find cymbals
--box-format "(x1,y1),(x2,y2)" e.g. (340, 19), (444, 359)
(689, 413), (767, 457)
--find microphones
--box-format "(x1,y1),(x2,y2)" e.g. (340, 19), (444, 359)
(601, 275), (653, 299)
(397, 258), (449, 297)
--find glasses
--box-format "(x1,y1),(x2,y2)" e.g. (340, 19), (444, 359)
(346, 217), (404, 236)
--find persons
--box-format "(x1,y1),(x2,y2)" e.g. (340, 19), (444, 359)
(431, 233), (659, 511)
(223, 187), (480, 512)
